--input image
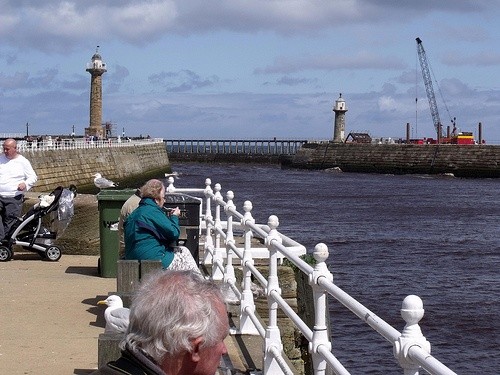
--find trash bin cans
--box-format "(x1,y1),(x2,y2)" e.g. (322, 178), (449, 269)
(163, 193), (201, 269)
(97, 190), (136, 278)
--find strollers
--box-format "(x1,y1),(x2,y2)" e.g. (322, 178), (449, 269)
(0, 185), (77, 262)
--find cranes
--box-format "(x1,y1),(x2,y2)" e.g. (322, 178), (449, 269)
(415, 36), (475, 143)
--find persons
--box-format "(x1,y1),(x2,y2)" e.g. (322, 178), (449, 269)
(24, 134), (129, 148)
(0, 138), (37, 245)
(118, 179), (205, 283)
(89, 269), (232, 375)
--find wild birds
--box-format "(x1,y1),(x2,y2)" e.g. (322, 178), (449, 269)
(96, 294), (131, 334)
(92, 173), (119, 190)
